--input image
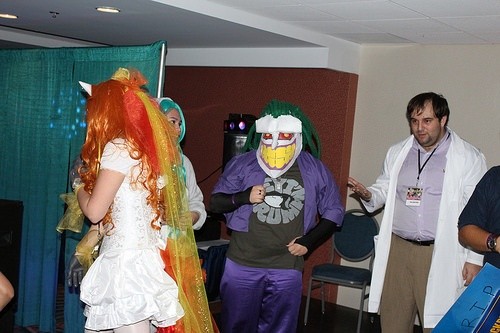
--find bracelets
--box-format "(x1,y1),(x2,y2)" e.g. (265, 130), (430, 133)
(232, 192), (242, 207)
(87, 228), (105, 237)
(486, 234), (493, 250)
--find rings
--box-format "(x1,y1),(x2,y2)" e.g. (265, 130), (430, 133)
(259, 190), (261, 194)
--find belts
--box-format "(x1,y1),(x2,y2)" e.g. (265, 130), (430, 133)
(397, 235), (434, 246)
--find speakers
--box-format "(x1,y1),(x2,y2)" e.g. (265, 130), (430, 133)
(0, 199), (24, 333)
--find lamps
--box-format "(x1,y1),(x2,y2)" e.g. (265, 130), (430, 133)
(223, 112), (256, 134)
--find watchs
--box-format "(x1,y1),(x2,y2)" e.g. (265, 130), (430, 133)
(488, 234), (499, 251)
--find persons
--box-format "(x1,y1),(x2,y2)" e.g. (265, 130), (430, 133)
(349, 92), (488, 333)
(210, 98), (345, 333)
(0, 272), (14, 311)
(56, 68), (214, 333)
(458, 164), (500, 270)
(407, 191), (422, 200)
(152, 98), (220, 333)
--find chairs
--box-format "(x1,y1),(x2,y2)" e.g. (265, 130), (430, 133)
(305, 209), (380, 333)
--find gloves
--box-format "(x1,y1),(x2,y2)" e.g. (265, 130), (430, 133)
(66, 252), (84, 287)
